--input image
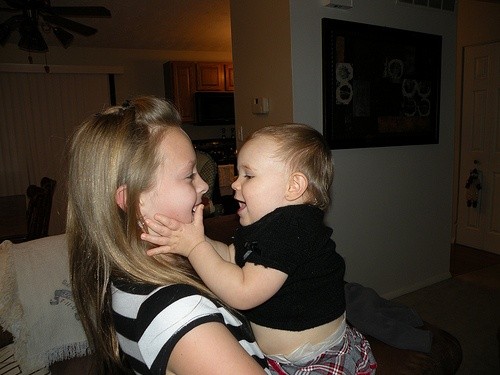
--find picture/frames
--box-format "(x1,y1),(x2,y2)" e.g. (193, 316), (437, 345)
(321, 18), (444, 150)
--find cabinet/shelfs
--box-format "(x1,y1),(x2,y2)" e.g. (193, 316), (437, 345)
(163, 60), (234, 123)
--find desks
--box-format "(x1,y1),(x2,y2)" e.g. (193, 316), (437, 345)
(0, 194), (28, 244)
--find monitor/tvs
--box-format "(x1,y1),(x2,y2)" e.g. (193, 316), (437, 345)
(321, 15), (444, 150)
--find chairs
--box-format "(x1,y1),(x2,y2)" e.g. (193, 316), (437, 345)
(26, 177), (57, 240)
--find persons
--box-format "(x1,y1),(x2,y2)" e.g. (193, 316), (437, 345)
(141, 123), (377, 375)
(48, 95), (271, 375)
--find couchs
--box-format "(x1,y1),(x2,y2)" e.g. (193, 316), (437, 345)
(0, 213), (465, 375)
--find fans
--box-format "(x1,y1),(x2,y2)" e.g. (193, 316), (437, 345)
(0, 0), (114, 53)
